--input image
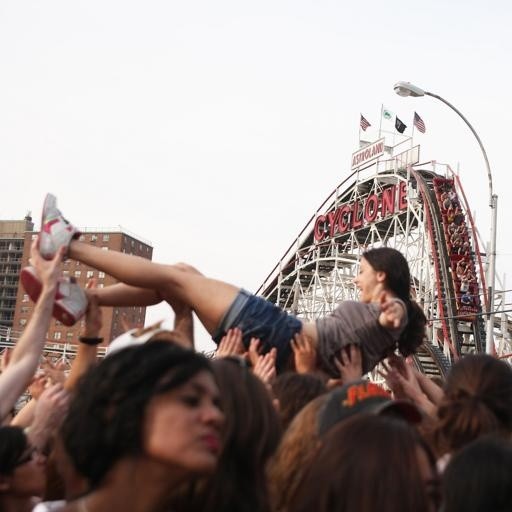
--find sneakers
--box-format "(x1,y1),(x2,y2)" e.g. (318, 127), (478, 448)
(19, 266), (89, 326)
(38, 194), (82, 260)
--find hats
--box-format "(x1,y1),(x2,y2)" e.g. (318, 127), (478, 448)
(105, 327), (189, 360)
(319, 380), (421, 440)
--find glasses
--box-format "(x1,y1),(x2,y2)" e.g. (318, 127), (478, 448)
(5, 445), (39, 471)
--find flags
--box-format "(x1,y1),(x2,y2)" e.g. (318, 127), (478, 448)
(414, 113), (426, 134)
(360, 116), (371, 131)
(382, 108), (393, 120)
(395, 118), (406, 134)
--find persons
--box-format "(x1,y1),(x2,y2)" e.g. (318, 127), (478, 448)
(440, 177), (474, 306)
(1, 234), (511, 512)
(21, 193), (427, 380)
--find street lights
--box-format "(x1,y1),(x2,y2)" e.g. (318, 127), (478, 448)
(394, 80), (499, 356)
